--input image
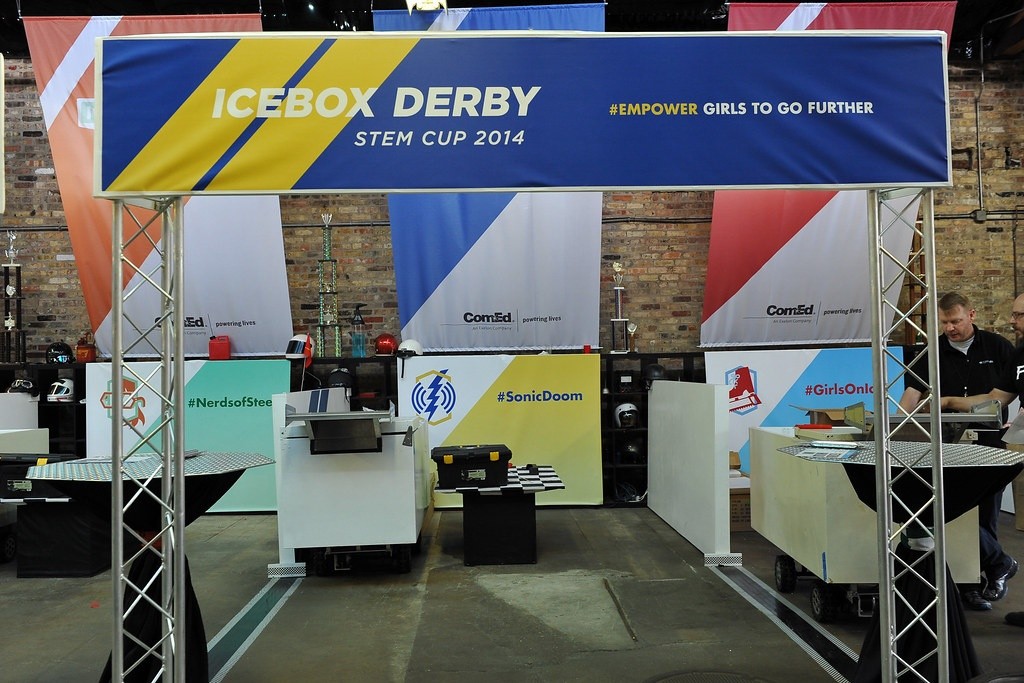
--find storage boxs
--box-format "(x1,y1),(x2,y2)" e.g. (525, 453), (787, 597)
(430, 444), (513, 490)
(0, 453), (79, 501)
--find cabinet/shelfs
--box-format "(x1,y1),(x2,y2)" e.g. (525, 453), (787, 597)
(601, 351), (705, 511)
(0, 361), (86, 455)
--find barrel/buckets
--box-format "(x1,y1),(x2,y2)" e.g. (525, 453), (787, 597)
(208, 336), (231, 360)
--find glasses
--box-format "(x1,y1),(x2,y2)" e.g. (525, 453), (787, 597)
(1012, 312), (1024, 320)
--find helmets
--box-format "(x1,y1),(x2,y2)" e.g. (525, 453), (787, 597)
(374, 333), (398, 356)
(620, 439), (640, 462)
(47, 378), (73, 402)
(641, 363), (669, 391)
(398, 339), (423, 356)
(614, 404), (639, 428)
(46, 342), (73, 365)
(329, 367), (353, 387)
(285, 333), (316, 359)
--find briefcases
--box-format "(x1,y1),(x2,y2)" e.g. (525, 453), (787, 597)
(431, 444), (512, 489)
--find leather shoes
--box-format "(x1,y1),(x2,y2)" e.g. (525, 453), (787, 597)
(963, 591), (992, 611)
(981, 555), (1018, 600)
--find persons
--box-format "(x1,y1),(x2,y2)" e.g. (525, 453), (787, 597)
(898, 292), (1019, 611)
(1003, 293), (1024, 627)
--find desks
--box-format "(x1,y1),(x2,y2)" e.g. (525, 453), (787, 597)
(435, 464), (564, 567)
(24, 449), (275, 683)
(777, 441), (1024, 683)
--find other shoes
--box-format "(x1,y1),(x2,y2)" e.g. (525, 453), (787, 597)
(1005, 612), (1024, 627)
